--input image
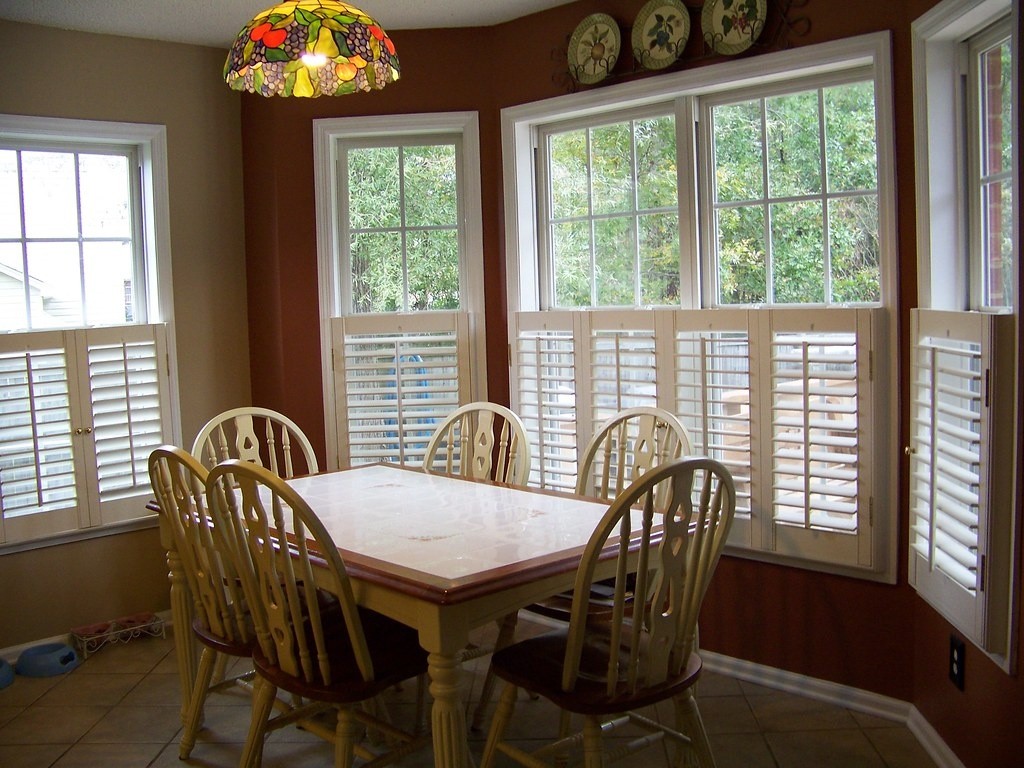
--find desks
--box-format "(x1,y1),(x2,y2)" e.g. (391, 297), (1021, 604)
(146, 463), (719, 768)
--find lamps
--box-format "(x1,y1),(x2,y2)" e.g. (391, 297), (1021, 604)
(222, 0), (400, 99)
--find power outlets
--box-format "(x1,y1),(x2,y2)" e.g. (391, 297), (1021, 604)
(949, 634), (966, 694)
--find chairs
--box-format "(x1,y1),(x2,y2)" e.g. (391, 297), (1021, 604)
(480, 457), (736, 768)
(423, 402), (539, 702)
(191, 398), (319, 686)
(206, 458), (430, 768)
(470, 406), (694, 733)
(148, 444), (340, 768)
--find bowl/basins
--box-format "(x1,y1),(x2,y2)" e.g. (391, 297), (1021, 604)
(0, 656), (14, 689)
(16, 644), (78, 678)
